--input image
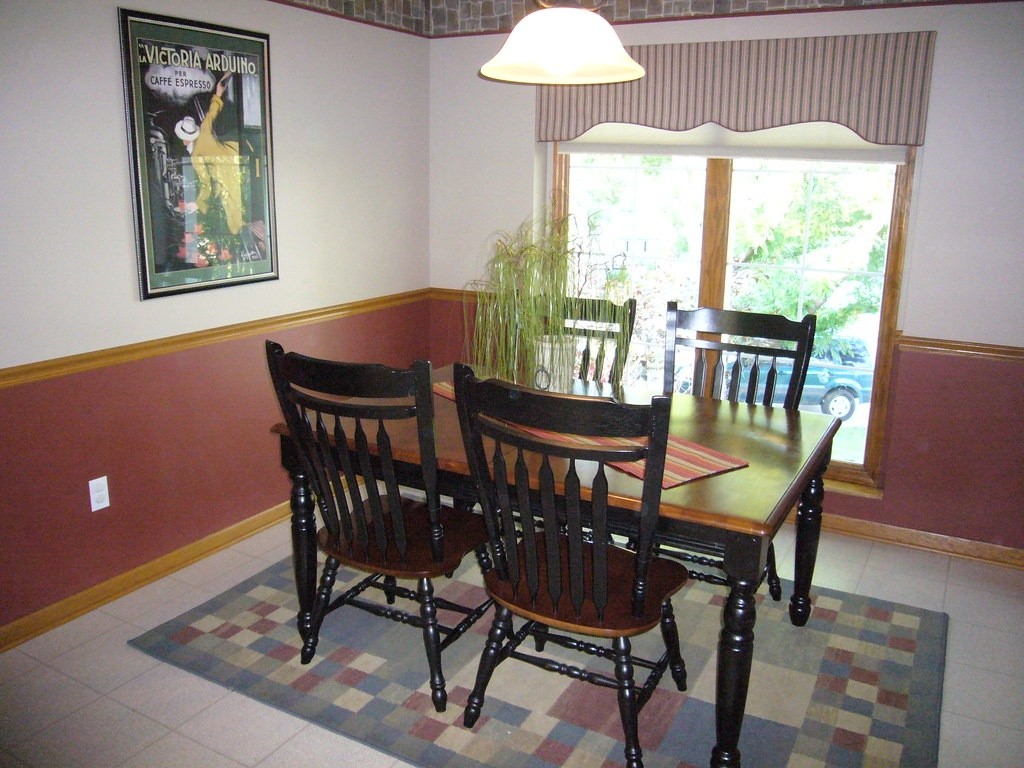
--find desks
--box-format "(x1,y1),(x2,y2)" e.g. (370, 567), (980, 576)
(269, 360), (840, 768)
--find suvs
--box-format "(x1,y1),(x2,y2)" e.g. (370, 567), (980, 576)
(677, 337), (876, 419)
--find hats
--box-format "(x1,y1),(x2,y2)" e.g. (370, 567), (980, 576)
(174, 116), (200, 141)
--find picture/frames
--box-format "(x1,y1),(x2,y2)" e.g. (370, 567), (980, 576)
(116, 7), (279, 301)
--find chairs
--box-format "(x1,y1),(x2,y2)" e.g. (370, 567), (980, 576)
(504, 289), (637, 384)
(451, 361), (689, 768)
(264, 339), (515, 713)
(662, 300), (817, 602)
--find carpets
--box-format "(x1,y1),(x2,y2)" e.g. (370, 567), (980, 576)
(125, 492), (950, 767)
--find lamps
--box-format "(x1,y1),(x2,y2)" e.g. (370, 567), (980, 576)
(477, 0), (647, 85)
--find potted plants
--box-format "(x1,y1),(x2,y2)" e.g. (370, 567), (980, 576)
(461, 185), (635, 405)
